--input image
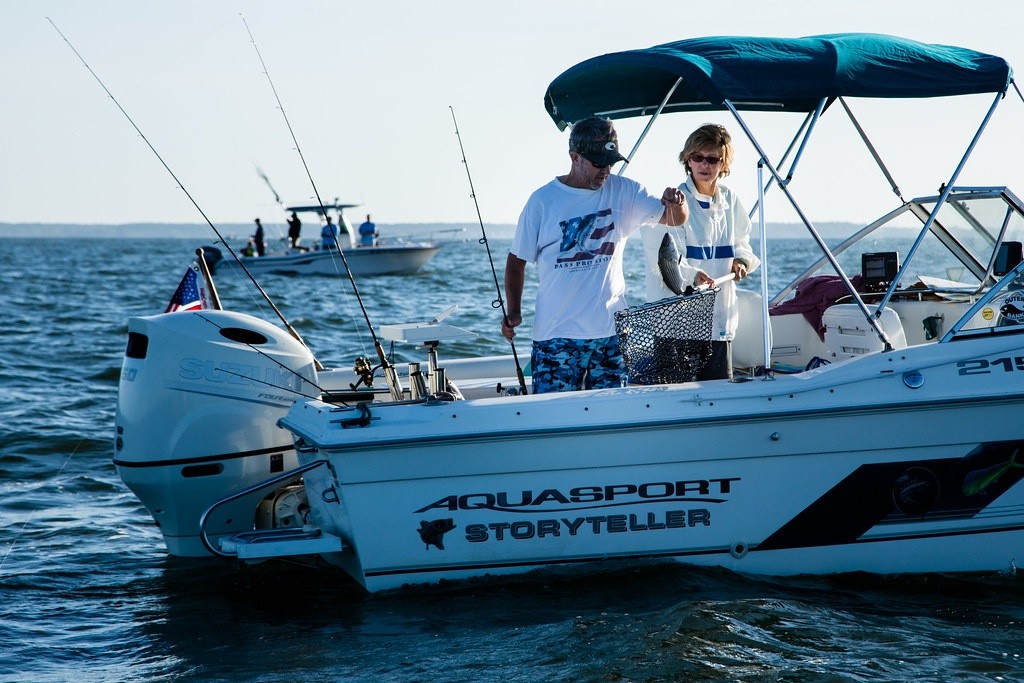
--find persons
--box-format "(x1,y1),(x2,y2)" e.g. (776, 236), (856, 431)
(359, 214), (376, 246)
(195, 246), (222, 276)
(287, 213), (309, 252)
(321, 217), (340, 250)
(502, 117), (689, 395)
(251, 218), (265, 256)
(641, 124), (761, 383)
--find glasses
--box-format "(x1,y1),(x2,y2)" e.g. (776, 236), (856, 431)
(690, 154), (723, 164)
(580, 153), (615, 169)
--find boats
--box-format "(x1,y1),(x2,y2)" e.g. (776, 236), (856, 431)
(200, 204), (444, 277)
(111, 32), (1024, 592)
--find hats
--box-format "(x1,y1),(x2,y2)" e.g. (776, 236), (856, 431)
(568, 138), (630, 165)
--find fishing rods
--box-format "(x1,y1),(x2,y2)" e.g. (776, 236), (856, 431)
(245, 16), (392, 391)
(443, 73), (529, 396)
(46, 15), (326, 371)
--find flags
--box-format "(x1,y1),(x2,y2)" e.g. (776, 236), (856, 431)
(169, 263), (210, 312)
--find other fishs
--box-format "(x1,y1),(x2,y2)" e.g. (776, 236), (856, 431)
(657, 230), (687, 296)
